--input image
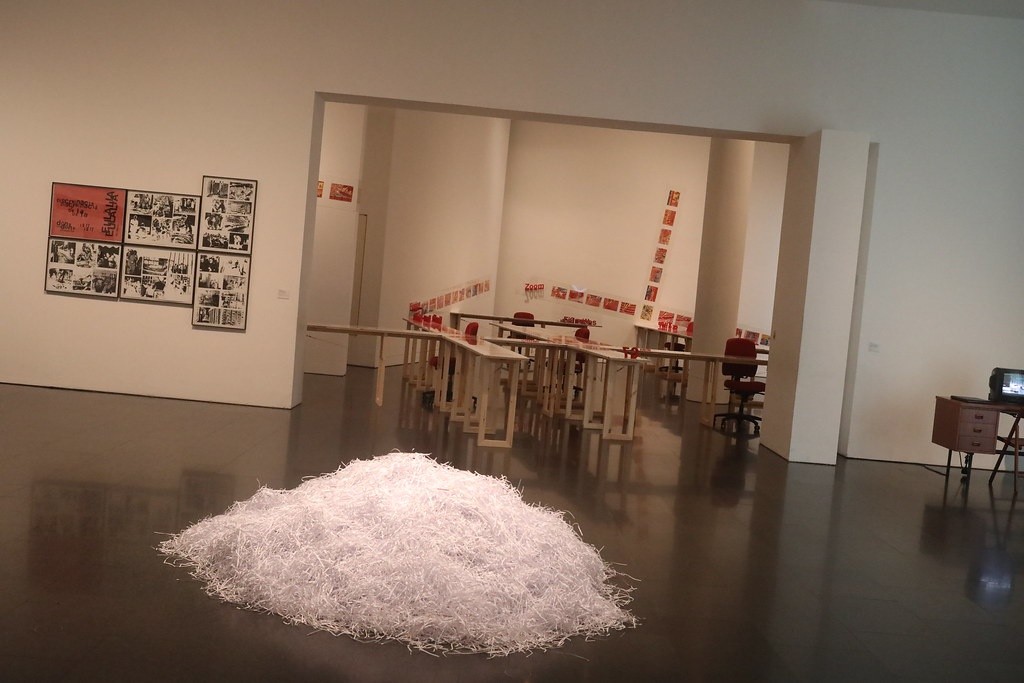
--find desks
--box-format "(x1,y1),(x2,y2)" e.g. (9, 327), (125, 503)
(931, 395), (1024, 478)
(308, 312), (769, 448)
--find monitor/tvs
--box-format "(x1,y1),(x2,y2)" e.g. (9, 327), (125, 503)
(988, 367), (1024, 403)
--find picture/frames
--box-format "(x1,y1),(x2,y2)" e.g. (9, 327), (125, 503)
(43, 174), (259, 332)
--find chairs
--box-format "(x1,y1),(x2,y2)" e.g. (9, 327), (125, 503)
(658, 321), (693, 373)
(989, 408), (1024, 495)
(506, 312), (535, 370)
(545, 328), (590, 394)
(421, 321), (479, 410)
(713, 338), (766, 437)
